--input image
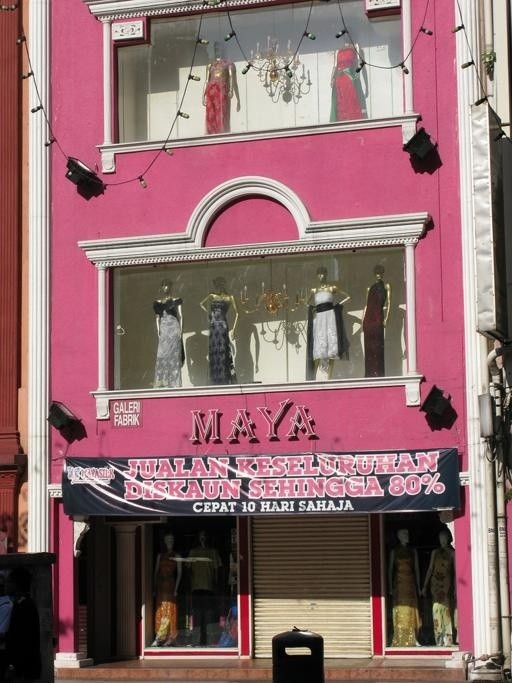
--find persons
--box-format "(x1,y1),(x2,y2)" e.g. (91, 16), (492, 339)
(154, 277), (185, 388)
(149, 530), (182, 648)
(182, 528), (222, 646)
(386, 527), (422, 646)
(361, 263), (391, 378)
(0, 570), (14, 682)
(421, 528), (458, 650)
(4, 568), (43, 683)
(304, 265), (351, 382)
(198, 275), (239, 386)
(329, 26), (369, 123)
(202, 40), (237, 134)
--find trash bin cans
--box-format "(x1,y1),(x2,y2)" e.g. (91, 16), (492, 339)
(272, 628), (324, 682)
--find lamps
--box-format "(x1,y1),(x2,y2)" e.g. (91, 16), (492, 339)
(418, 384), (453, 418)
(400, 126), (437, 160)
(46, 399), (78, 434)
(246, 9), (301, 81)
(64, 153), (99, 191)
(237, 258), (317, 318)
